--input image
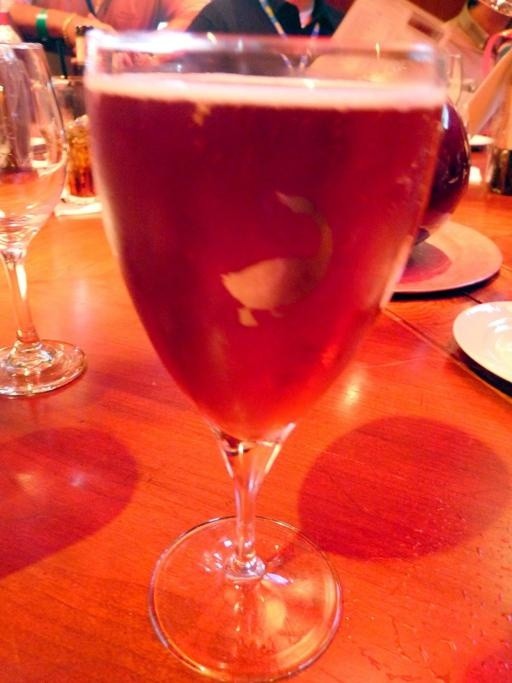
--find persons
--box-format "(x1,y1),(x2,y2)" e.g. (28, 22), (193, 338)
(161, 0), (346, 78)
(8, 0), (210, 74)
(444, 0), (512, 60)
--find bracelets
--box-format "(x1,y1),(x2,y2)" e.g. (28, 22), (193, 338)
(62, 13), (78, 47)
(35, 6), (49, 41)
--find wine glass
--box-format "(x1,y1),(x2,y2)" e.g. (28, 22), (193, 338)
(0, 42), (87, 395)
(86, 29), (468, 683)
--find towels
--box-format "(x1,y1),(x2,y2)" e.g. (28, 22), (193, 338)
(52, 189), (101, 217)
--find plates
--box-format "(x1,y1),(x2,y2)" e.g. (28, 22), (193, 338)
(452, 301), (510, 381)
(390, 221), (504, 295)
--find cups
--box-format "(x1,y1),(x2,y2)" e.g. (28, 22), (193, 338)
(42, 71), (109, 221)
